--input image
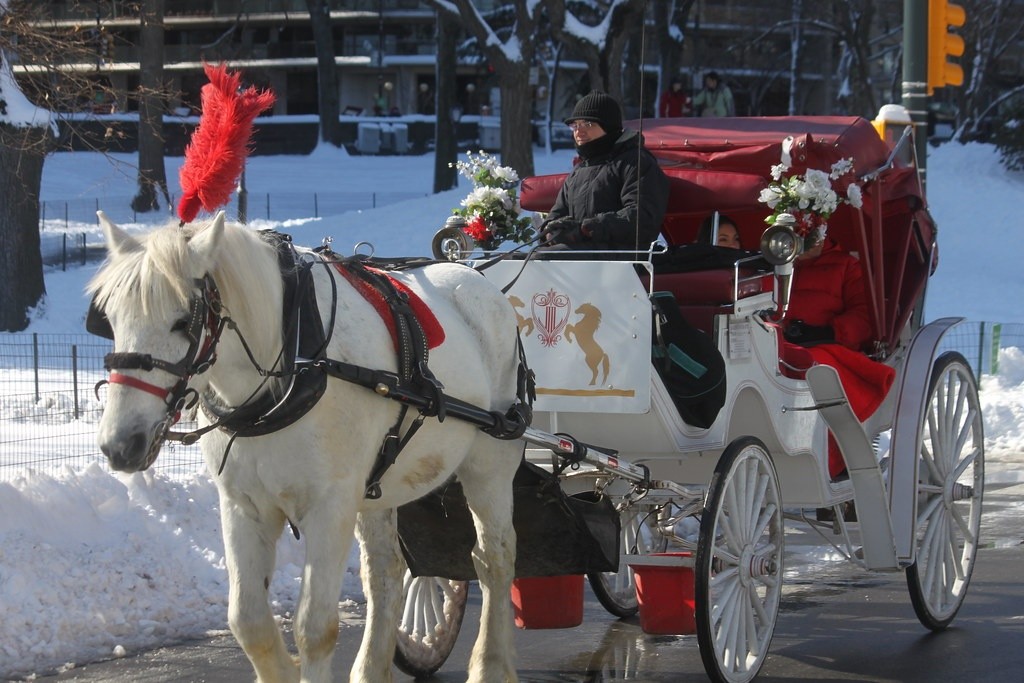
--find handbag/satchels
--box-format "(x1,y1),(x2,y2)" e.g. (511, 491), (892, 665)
(701, 108), (718, 117)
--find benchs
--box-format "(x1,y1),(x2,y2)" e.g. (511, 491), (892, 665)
(652, 247), (774, 327)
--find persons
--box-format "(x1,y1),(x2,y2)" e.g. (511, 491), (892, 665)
(373, 105), (385, 117)
(536, 92), (674, 277)
(658, 77), (691, 119)
(688, 70), (736, 119)
(388, 107), (401, 117)
(695, 216), (741, 254)
(766, 230), (874, 347)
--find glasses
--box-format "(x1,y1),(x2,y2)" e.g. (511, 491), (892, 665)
(568, 121), (597, 129)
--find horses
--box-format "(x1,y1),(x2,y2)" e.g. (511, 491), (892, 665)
(80, 209), (527, 683)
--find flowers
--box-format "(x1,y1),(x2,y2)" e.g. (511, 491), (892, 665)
(450, 149), (534, 257)
(773, 128), (858, 258)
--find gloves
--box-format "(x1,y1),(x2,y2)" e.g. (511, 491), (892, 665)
(547, 215), (586, 246)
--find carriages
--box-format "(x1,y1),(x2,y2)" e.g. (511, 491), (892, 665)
(75, 111), (988, 683)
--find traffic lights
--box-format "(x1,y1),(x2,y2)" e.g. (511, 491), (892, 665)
(927, 0), (966, 97)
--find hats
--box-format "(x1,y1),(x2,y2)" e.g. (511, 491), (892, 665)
(565, 89), (623, 157)
